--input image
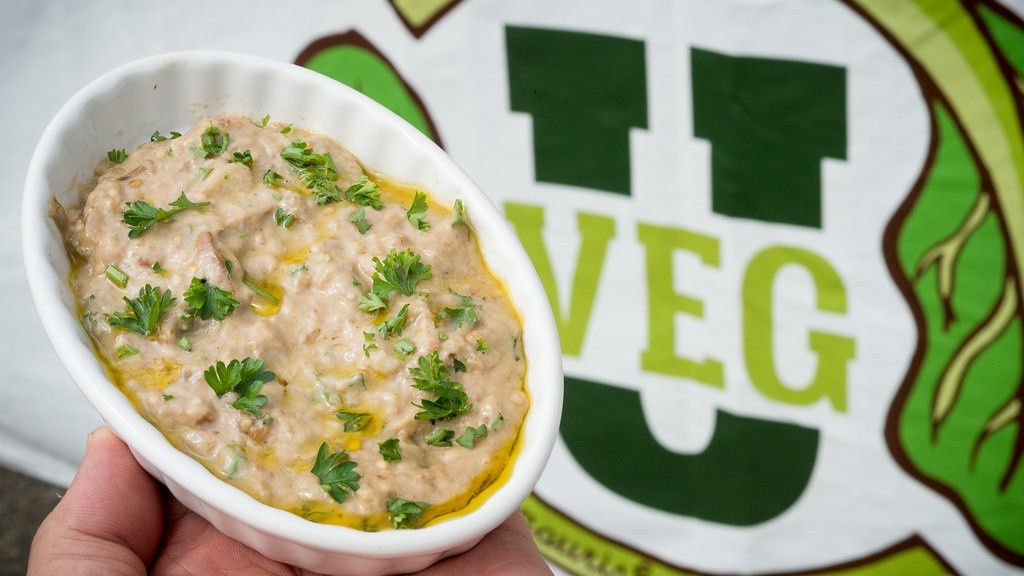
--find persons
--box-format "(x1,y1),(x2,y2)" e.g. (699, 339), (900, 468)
(26, 427), (551, 576)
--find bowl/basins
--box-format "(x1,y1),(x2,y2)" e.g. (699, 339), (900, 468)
(20, 50), (565, 576)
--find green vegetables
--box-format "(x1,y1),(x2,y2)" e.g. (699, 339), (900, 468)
(91, 113), (506, 530)
